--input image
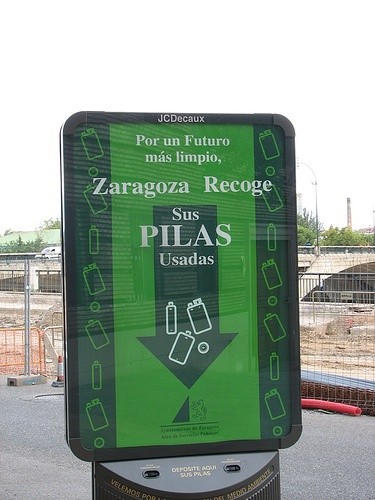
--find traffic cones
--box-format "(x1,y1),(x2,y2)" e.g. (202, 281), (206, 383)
(51, 355), (63, 387)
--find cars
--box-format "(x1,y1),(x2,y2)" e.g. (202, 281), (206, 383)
(34, 247), (61, 260)
